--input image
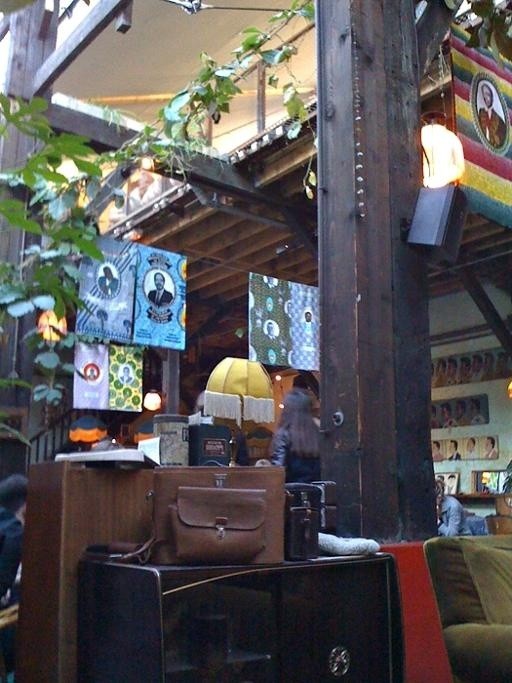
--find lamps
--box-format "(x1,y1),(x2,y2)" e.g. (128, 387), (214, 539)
(202, 358), (275, 466)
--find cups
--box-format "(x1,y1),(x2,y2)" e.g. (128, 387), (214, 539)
(153, 413), (189, 468)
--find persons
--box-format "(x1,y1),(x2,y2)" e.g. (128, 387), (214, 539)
(428, 351), (511, 492)
(301, 312), (315, 332)
(267, 322), (276, 338)
(147, 272), (172, 308)
(437, 479), (472, 540)
(478, 83), (506, 147)
(99, 264), (117, 294)
(0, 474), (30, 608)
(272, 387), (321, 481)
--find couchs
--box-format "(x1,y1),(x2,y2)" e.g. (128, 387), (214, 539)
(422, 533), (511, 683)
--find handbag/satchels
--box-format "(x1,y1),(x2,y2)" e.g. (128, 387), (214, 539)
(151, 467), (285, 567)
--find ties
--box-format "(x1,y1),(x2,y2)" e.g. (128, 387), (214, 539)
(155, 292), (160, 303)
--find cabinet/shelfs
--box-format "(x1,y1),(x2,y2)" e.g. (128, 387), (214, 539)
(79, 552), (403, 683)
